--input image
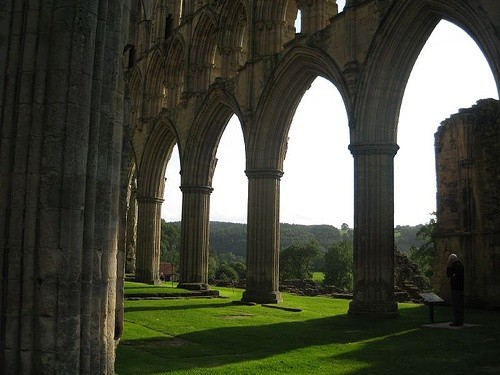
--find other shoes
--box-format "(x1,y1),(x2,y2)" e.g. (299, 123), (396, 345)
(449, 321), (463, 326)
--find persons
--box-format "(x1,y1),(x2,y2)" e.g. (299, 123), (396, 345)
(446, 254), (466, 326)
